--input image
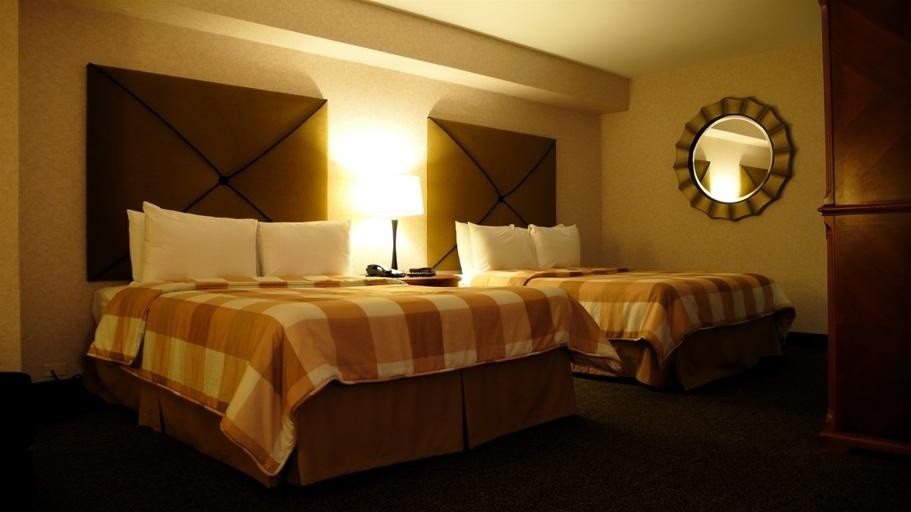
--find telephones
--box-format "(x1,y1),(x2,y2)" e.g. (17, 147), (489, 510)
(367, 264), (405, 277)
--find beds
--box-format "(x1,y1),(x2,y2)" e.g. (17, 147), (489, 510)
(463, 266), (773, 392)
(92, 274), (569, 486)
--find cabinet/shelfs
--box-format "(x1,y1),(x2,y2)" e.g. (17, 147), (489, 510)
(816, 1), (909, 452)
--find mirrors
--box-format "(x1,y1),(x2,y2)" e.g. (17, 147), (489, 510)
(675, 96), (795, 220)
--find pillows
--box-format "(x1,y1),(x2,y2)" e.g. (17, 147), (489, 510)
(126, 200), (350, 281)
(456, 222), (581, 273)
